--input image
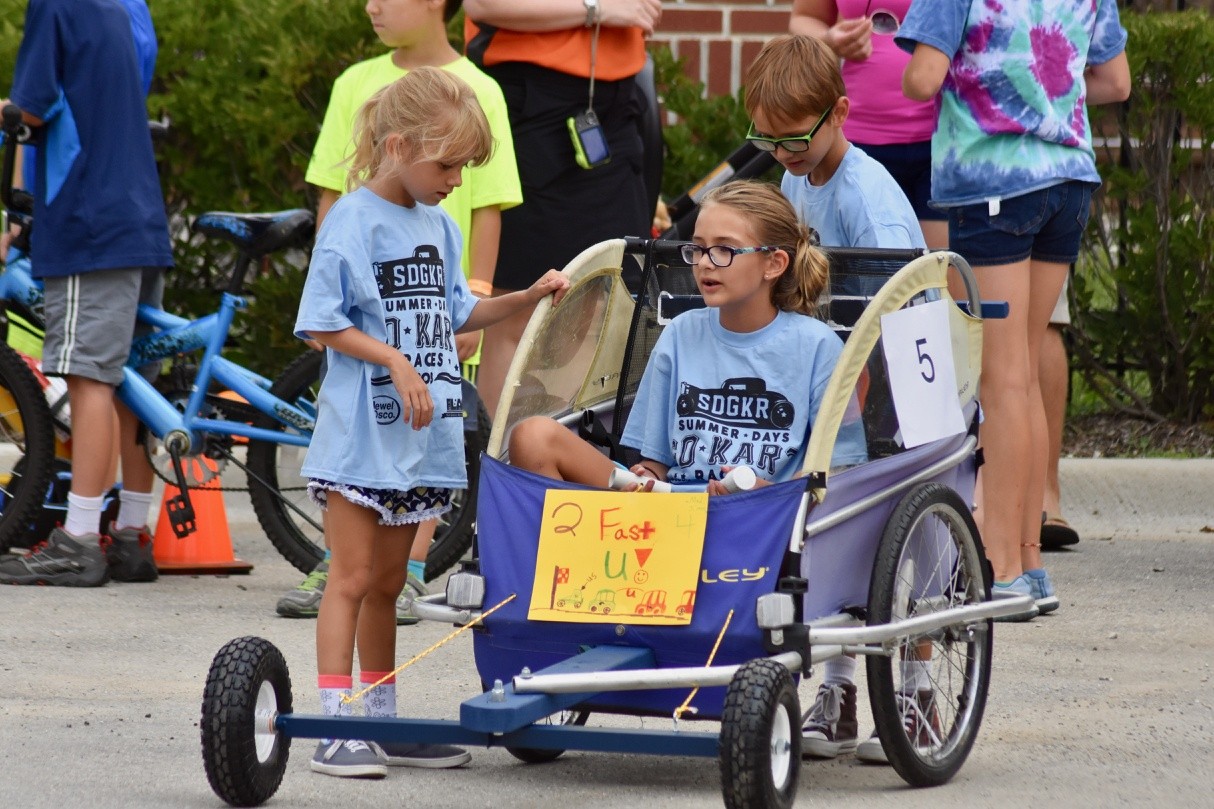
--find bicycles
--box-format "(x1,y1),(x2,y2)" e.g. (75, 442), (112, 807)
(0, 106), (493, 585)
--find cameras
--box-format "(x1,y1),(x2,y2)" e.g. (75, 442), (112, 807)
(567, 111), (611, 170)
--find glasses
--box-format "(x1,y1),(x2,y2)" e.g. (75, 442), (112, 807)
(679, 242), (774, 267)
(869, 10), (901, 35)
(744, 109), (831, 152)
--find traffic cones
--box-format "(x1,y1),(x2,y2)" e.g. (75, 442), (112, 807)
(147, 407), (253, 577)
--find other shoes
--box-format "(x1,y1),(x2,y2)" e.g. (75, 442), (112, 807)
(1042, 513), (1081, 545)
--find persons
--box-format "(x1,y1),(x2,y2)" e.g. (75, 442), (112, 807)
(457, 0), (661, 435)
(270, 0), (521, 626)
(786, 1), (950, 254)
(902, 0), (1131, 615)
(1039, 278), (1081, 549)
(0, 0), (168, 584)
(743, 35), (942, 763)
(306, 67), (571, 778)
(509, 178), (867, 494)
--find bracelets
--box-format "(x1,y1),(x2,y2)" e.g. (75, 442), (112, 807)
(468, 279), (492, 296)
(583, 0), (595, 29)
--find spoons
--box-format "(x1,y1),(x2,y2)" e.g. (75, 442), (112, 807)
(868, 10), (900, 36)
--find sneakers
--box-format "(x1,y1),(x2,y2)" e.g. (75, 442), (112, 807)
(106, 522), (160, 581)
(856, 690), (943, 762)
(993, 577), (1040, 622)
(0, 522), (105, 586)
(800, 683), (858, 754)
(393, 571), (430, 624)
(376, 735), (471, 768)
(274, 553), (333, 617)
(1026, 568), (1063, 611)
(312, 734), (389, 778)
